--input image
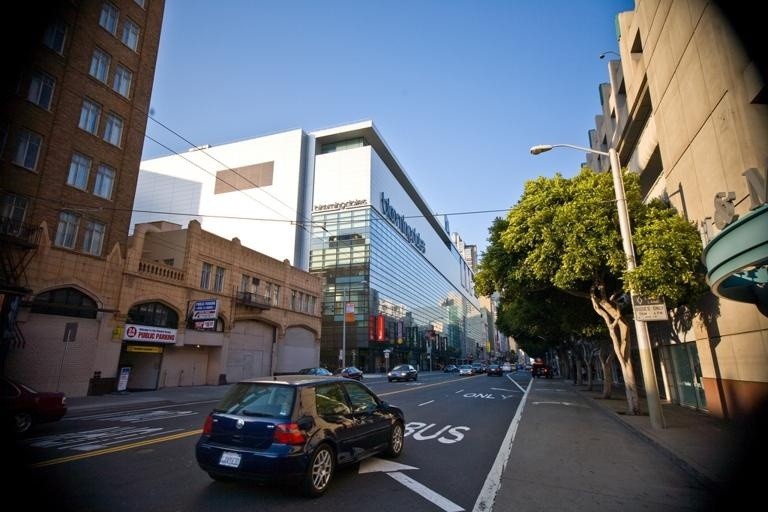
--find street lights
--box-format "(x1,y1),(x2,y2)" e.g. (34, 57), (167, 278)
(530, 144), (666, 431)
(429, 317), (444, 372)
(342, 281), (368, 367)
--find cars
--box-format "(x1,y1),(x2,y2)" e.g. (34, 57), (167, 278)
(0, 374), (69, 436)
(444, 362), (532, 377)
(531, 362), (554, 379)
(297, 367), (364, 382)
(195, 374), (406, 497)
(387, 364), (418, 382)
